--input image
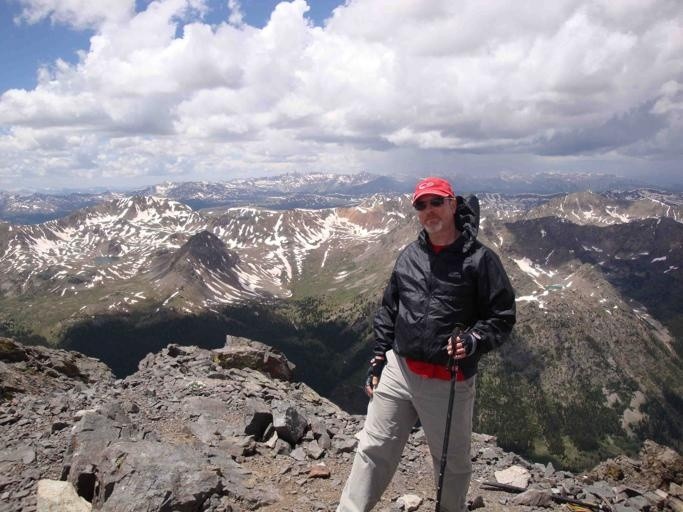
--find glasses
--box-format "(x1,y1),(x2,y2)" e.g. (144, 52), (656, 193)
(414, 197), (456, 211)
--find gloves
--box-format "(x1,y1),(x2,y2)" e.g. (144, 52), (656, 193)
(365, 360), (384, 386)
(459, 334), (473, 356)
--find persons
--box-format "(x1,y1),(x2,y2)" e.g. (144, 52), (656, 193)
(335, 177), (516, 511)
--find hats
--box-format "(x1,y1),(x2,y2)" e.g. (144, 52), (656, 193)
(411, 177), (454, 203)
